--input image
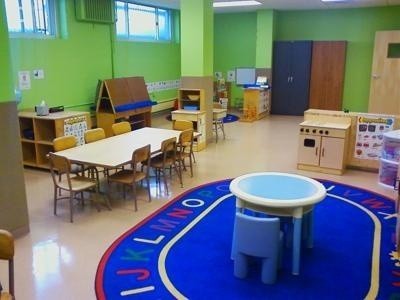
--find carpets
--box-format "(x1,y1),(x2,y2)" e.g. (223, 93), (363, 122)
(93, 173), (400, 300)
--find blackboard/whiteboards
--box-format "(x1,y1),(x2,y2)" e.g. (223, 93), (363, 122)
(237, 69), (256, 84)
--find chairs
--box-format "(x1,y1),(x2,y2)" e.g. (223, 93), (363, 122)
(233, 213), (285, 285)
(45, 119), (203, 223)
(212, 102), (227, 143)
(0, 228), (17, 300)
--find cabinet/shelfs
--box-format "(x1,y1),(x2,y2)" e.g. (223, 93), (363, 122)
(18, 75), (157, 174)
(269, 40), (346, 115)
(296, 108), (400, 176)
(170, 88), (207, 152)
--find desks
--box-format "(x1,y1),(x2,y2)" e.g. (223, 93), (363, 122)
(230, 171), (327, 275)
